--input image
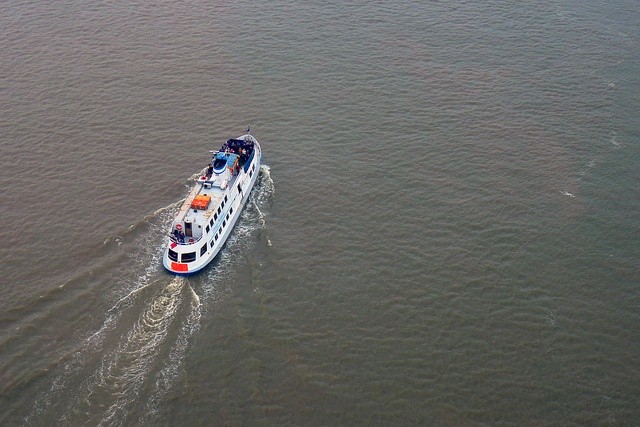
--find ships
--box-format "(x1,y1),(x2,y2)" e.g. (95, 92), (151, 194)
(162, 122), (261, 273)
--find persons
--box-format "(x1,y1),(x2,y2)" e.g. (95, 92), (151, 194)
(219, 141), (252, 161)
(179, 231), (185, 242)
(236, 163), (240, 176)
(208, 164), (213, 177)
